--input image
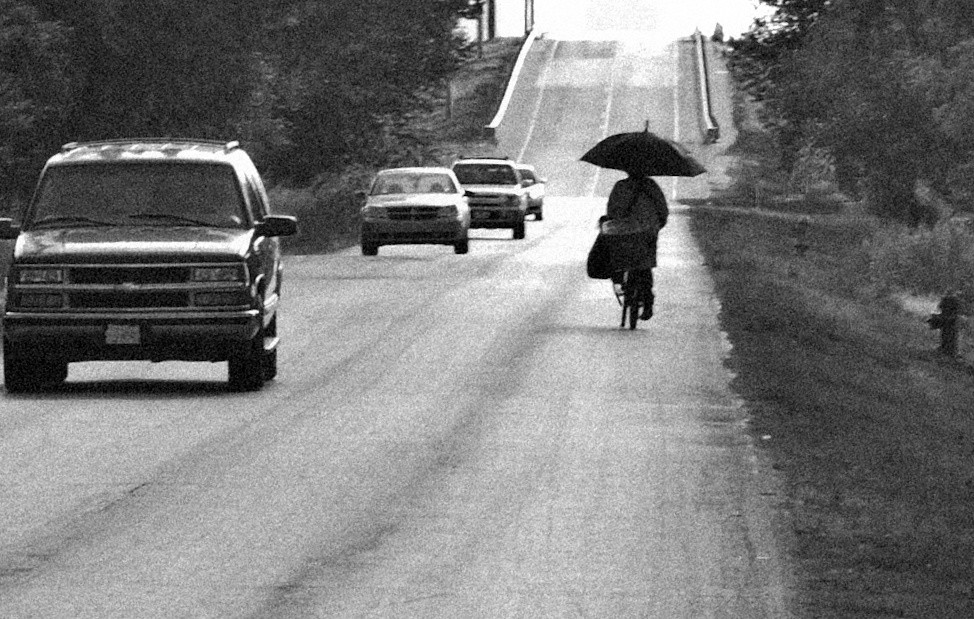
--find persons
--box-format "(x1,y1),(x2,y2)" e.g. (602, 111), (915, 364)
(606, 171), (670, 320)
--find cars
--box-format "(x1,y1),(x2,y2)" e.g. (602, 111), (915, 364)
(511, 163), (545, 220)
(356, 168), (475, 257)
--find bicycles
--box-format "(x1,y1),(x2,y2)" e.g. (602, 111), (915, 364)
(603, 214), (640, 327)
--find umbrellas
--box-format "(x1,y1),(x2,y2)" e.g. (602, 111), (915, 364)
(577, 119), (709, 181)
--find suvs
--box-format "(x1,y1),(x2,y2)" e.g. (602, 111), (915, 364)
(452, 154), (527, 240)
(0, 136), (299, 391)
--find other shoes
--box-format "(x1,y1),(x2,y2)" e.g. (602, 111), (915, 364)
(612, 271), (624, 284)
(643, 302), (653, 320)
